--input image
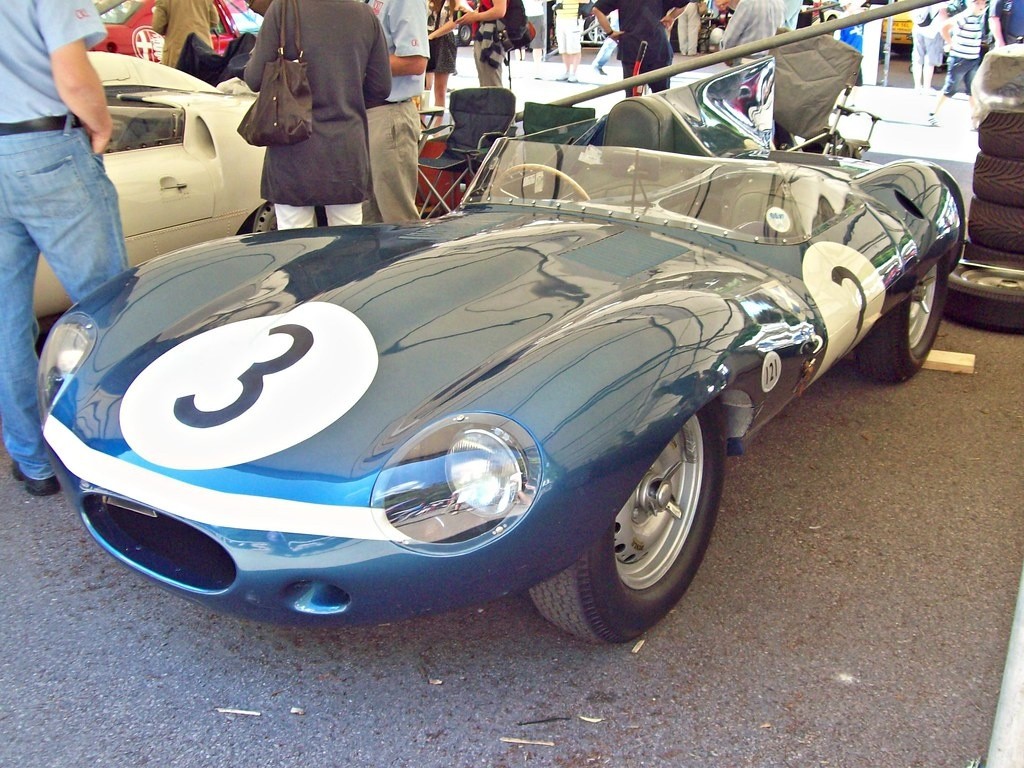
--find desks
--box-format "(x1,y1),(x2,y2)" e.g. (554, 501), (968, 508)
(419, 104), (445, 157)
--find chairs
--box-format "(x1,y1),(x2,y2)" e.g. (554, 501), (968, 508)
(724, 182), (824, 239)
(416, 86), (516, 221)
(583, 97), (673, 200)
(769, 27), (883, 160)
(467, 101), (598, 198)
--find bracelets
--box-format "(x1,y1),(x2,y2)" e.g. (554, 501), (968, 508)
(606, 30), (613, 36)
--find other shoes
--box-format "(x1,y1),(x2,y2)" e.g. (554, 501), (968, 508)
(927, 111), (941, 128)
(923, 86), (936, 95)
(592, 64), (606, 76)
(12, 460), (59, 496)
(556, 74), (568, 81)
(568, 76), (578, 83)
(914, 88), (924, 95)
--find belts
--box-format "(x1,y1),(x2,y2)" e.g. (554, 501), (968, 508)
(0, 114), (82, 136)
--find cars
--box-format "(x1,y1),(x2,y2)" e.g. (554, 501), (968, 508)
(708, 4), (845, 46)
(27, 52), (970, 647)
(34, 50), (281, 330)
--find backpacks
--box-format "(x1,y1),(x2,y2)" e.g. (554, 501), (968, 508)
(482, 0), (536, 51)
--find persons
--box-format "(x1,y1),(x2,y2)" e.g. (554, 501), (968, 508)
(715, 0), (803, 68)
(591, 9), (619, 77)
(592, 0), (690, 97)
(0, 0), (129, 496)
(244, 0), (392, 229)
(678, 0), (703, 55)
(421, 0), (461, 137)
(555, 0), (582, 83)
(910, 0), (1024, 132)
(354, 0), (430, 224)
(152, 0), (220, 69)
(515, 0), (546, 62)
(455, 0), (507, 86)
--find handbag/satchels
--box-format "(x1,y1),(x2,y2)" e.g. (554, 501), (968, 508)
(237, 0), (312, 145)
